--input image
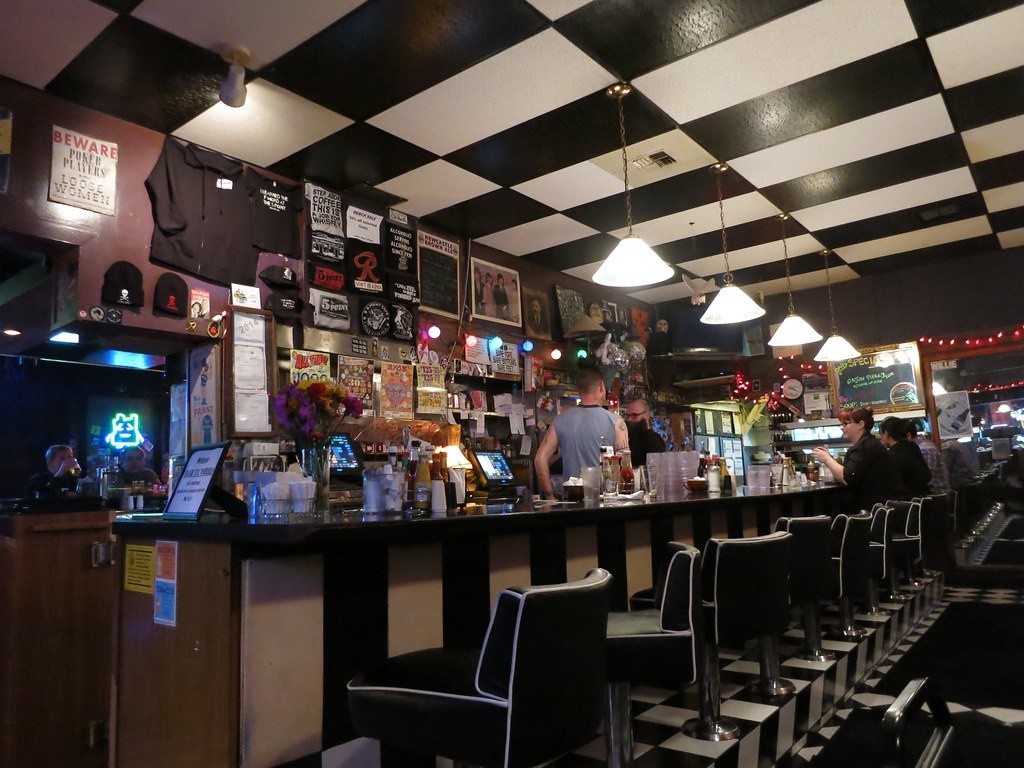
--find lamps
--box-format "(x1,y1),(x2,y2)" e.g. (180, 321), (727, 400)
(566, 317), (606, 363)
(768, 213), (823, 347)
(699, 160), (766, 325)
(812, 250), (862, 362)
(217, 45), (253, 108)
(593, 84), (674, 289)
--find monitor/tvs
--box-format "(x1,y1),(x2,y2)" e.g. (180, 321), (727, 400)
(328, 432), (364, 475)
(473, 449), (521, 486)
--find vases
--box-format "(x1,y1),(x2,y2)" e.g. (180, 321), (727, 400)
(296, 446), (330, 512)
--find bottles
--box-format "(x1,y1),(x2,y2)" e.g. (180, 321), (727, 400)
(362, 440), (456, 515)
(774, 413), (793, 423)
(775, 432), (792, 441)
(807, 461), (817, 481)
(600, 448), (634, 503)
(784, 466), (789, 486)
(702, 451), (732, 493)
(951, 409), (969, 430)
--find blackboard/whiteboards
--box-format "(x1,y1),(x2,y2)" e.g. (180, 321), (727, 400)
(827, 341), (927, 418)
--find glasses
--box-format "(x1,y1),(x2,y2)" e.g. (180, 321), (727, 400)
(625, 411), (646, 420)
(842, 421), (857, 427)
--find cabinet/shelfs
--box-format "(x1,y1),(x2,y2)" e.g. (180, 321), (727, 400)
(771, 409), (926, 447)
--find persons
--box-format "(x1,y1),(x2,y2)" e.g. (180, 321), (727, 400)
(620, 398), (666, 492)
(875, 416), (952, 577)
(535, 369), (632, 511)
(812, 406), (894, 567)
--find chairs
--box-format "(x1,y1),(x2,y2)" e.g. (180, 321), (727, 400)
(348, 567), (612, 767)
(608, 489), (956, 743)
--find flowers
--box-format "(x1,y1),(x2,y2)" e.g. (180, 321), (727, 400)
(274, 379), (363, 510)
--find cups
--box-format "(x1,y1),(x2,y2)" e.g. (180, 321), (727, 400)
(647, 450), (700, 499)
(69, 458), (82, 475)
(581, 466), (601, 501)
(259, 484), (292, 517)
(748, 463), (784, 491)
(123, 495), (143, 510)
(289, 482), (318, 516)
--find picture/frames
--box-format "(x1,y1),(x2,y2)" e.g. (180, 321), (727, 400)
(469, 257), (523, 328)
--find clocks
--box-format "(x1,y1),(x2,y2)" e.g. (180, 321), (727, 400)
(782, 379), (804, 400)
